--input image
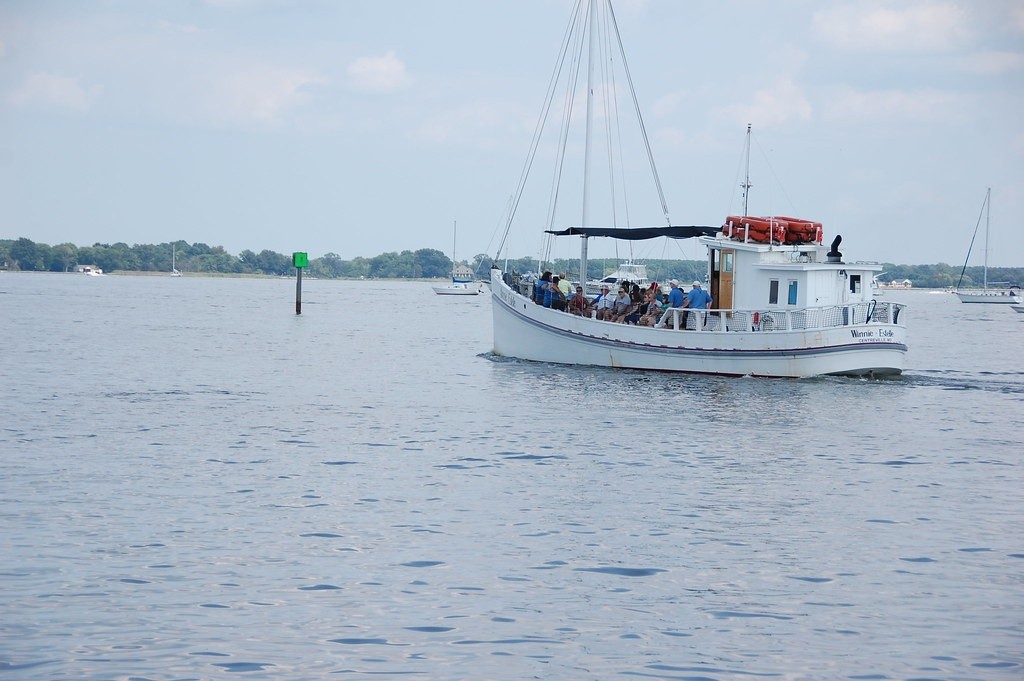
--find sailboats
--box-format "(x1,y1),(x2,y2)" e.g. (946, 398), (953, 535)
(952, 187), (1021, 304)
(488, 0), (908, 381)
(433, 220), (482, 296)
(171, 244), (183, 277)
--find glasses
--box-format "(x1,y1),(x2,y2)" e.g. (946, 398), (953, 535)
(577, 290), (582, 292)
(649, 297), (650, 299)
(663, 298), (665, 299)
(618, 291), (623, 293)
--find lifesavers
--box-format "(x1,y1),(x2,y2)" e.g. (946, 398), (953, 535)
(759, 314), (779, 331)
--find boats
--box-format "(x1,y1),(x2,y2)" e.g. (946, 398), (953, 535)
(1011, 306), (1024, 313)
(870, 277), (883, 296)
(945, 286), (958, 294)
(84, 267), (104, 277)
(569, 261), (650, 297)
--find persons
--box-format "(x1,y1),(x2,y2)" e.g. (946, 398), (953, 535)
(535, 272), (713, 330)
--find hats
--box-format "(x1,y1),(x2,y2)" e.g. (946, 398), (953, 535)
(553, 277), (560, 283)
(669, 279), (678, 285)
(560, 272), (565, 278)
(692, 280), (700, 285)
(600, 284), (609, 289)
